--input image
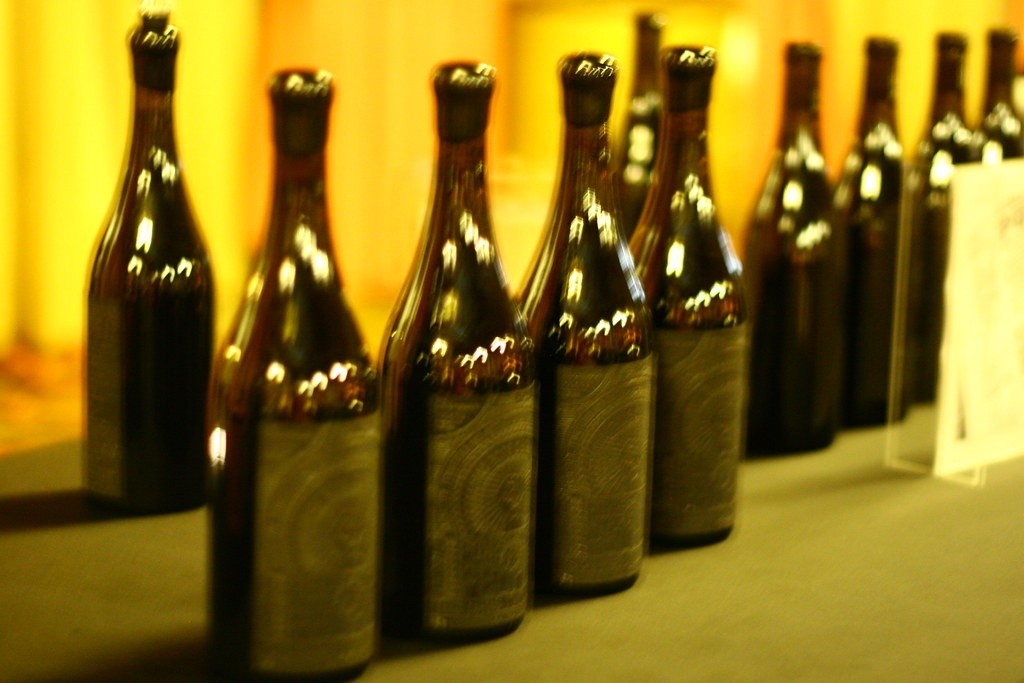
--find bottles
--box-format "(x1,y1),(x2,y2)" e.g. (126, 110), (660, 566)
(518, 47), (658, 606)
(740, 39), (847, 457)
(611, 9), (669, 239)
(629, 43), (750, 554)
(978, 29), (1024, 160)
(909, 31), (981, 406)
(834, 35), (921, 430)
(81, 4), (214, 516)
(376, 59), (535, 648)
(205, 66), (385, 682)
